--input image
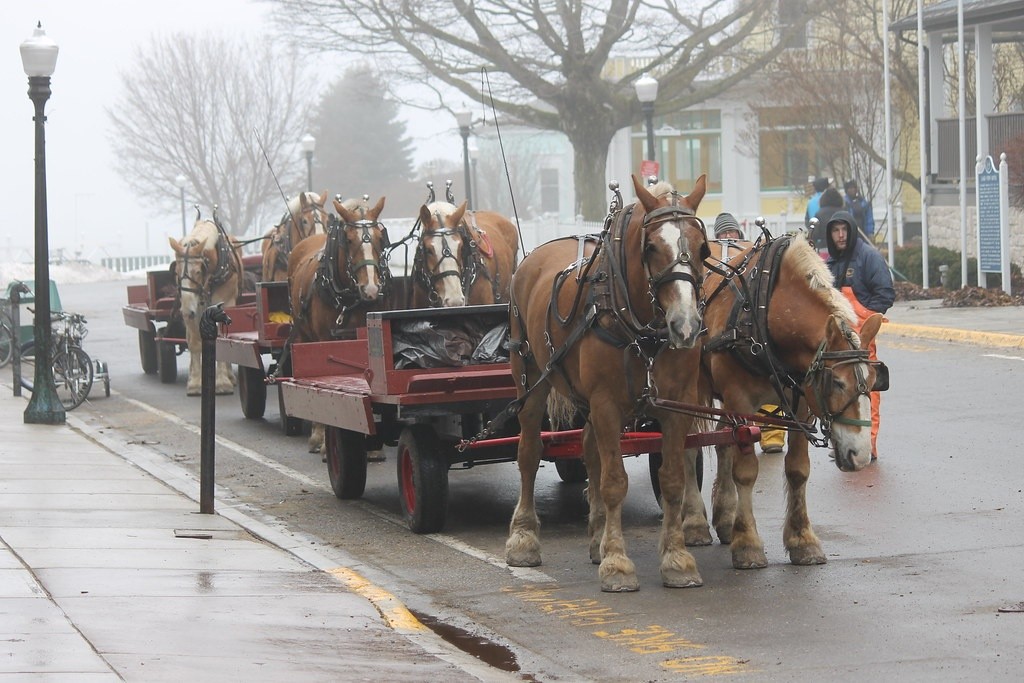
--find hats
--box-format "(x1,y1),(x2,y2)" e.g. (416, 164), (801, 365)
(813, 178), (829, 192)
(820, 187), (843, 208)
(714, 213), (742, 239)
(844, 179), (856, 192)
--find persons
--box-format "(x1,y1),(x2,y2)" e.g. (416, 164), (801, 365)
(825, 211), (896, 458)
(804, 177), (875, 262)
(715, 213), (787, 452)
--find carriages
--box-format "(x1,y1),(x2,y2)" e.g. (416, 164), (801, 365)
(122, 191), (332, 396)
(216, 178), (517, 464)
(261, 174), (888, 594)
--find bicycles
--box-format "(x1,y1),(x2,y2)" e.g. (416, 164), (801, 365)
(0, 305), (17, 368)
(16, 304), (111, 413)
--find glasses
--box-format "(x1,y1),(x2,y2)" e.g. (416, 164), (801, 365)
(720, 230), (738, 238)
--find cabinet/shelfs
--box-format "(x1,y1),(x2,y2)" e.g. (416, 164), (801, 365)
(367, 304), (513, 396)
(147, 256), (262, 310)
(257, 276), (414, 347)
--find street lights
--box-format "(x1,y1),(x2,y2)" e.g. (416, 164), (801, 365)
(16, 19), (68, 423)
(298, 131), (316, 187)
(455, 100), (474, 213)
(633, 71), (660, 161)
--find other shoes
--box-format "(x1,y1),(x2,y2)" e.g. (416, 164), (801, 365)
(766, 446), (782, 453)
(828, 451), (836, 460)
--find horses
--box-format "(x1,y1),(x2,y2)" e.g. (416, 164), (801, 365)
(287, 196), (386, 342)
(169, 220), (243, 396)
(260, 190), (329, 281)
(504, 174), (711, 592)
(680, 234), (884, 569)
(409, 202), (518, 310)
